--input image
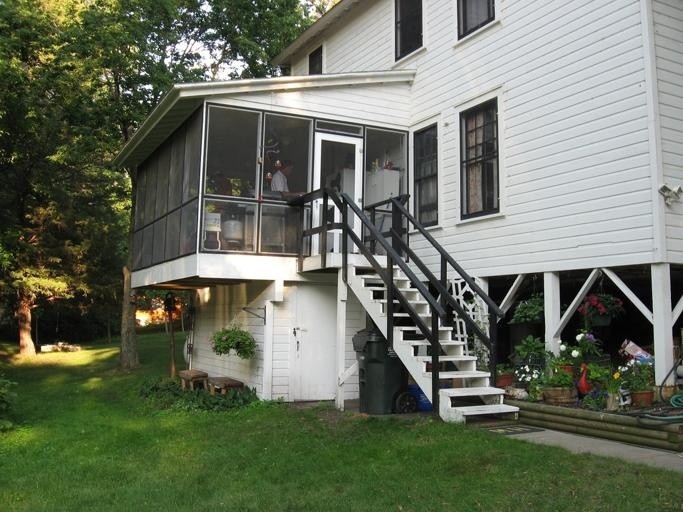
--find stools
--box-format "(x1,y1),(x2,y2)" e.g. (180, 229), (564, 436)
(178, 369), (208, 392)
(207, 377), (245, 396)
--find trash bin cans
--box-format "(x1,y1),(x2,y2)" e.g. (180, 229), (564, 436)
(352, 326), (418, 414)
(407, 382), (451, 411)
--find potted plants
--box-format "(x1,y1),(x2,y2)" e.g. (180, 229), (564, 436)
(496, 364), (513, 386)
(550, 357), (575, 376)
(507, 297), (544, 338)
(544, 376), (574, 403)
(626, 362), (654, 407)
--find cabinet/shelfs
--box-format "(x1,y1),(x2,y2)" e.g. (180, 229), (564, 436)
(367, 169), (399, 209)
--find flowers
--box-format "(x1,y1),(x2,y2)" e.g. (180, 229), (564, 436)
(586, 368), (625, 395)
(210, 327), (257, 359)
(577, 294), (627, 316)
(582, 388), (608, 409)
(515, 365), (544, 392)
(558, 329), (599, 359)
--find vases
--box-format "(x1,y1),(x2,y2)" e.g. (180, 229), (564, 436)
(607, 391), (623, 412)
(589, 314), (612, 328)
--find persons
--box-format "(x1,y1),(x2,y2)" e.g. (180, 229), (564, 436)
(271, 170), (290, 192)
(36, 342), (64, 353)
